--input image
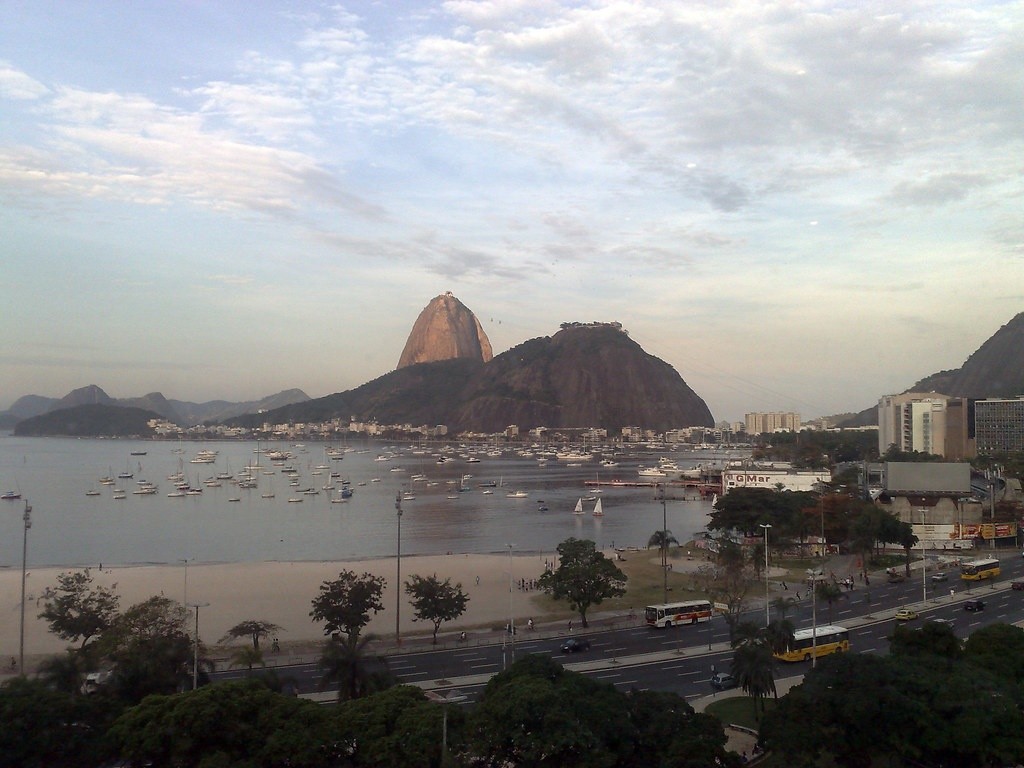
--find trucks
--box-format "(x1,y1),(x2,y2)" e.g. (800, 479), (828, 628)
(80, 671), (112, 696)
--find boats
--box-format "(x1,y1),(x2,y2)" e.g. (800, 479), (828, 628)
(86, 442), (753, 503)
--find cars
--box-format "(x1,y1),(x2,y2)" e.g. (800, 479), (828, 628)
(1012, 582), (1024, 590)
(711, 672), (738, 690)
(895, 610), (919, 621)
(560, 638), (590, 653)
(931, 572), (947, 581)
(964, 599), (986, 612)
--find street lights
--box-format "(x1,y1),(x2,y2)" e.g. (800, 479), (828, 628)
(760, 525), (772, 628)
(18, 499), (33, 677)
(429, 696), (468, 768)
(176, 557), (195, 609)
(186, 601), (209, 689)
(505, 543), (518, 663)
(918, 509), (929, 603)
(394, 491), (403, 642)
(806, 568), (823, 668)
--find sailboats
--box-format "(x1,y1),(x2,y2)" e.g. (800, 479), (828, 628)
(593, 498), (605, 516)
(574, 497), (583, 514)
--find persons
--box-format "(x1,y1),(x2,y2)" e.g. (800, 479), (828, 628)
(519, 562), (553, 592)
(840, 569), (870, 590)
(11, 656), (15, 671)
(476, 576), (480, 584)
(779, 583), (812, 599)
(886, 566), (890, 574)
(569, 621), (571, 631)
(630, 608), (634, 619)
(461, 631), (466, 642)
(528, 619), (534, 630)
(272, 639), (280, 653)
(951, 589), (954, 598)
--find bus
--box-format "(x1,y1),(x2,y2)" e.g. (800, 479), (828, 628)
(960, 558), (1000, 581)
(772, 625), (853, 662)
(643, 600), (713, 629)
